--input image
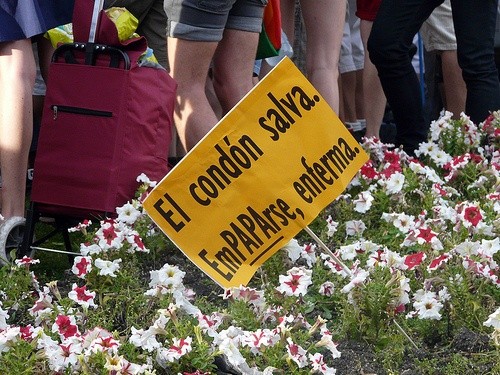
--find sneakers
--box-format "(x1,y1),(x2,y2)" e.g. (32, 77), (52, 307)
(357, 119), (367, 130)
(345, 121), (361, 132)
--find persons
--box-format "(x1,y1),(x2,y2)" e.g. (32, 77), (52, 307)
(164, 0), (268, 155)
(367, 0), (500, 159)
(0, 0), (223, 226)
(253, 0), (467, 145)
